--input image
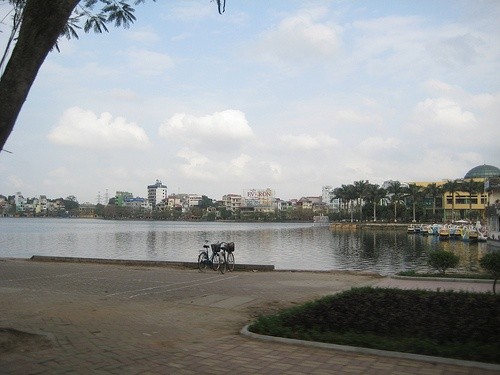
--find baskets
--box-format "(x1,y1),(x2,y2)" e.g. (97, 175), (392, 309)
(211, 243), (221, 252)
(224, 241), (234, 253)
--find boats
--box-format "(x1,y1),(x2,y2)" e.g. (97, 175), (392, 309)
(407, 220), (488, 242)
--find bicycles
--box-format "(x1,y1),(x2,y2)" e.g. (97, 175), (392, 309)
(219, 241), (235, 274)
(198, 239), (225, 273)
